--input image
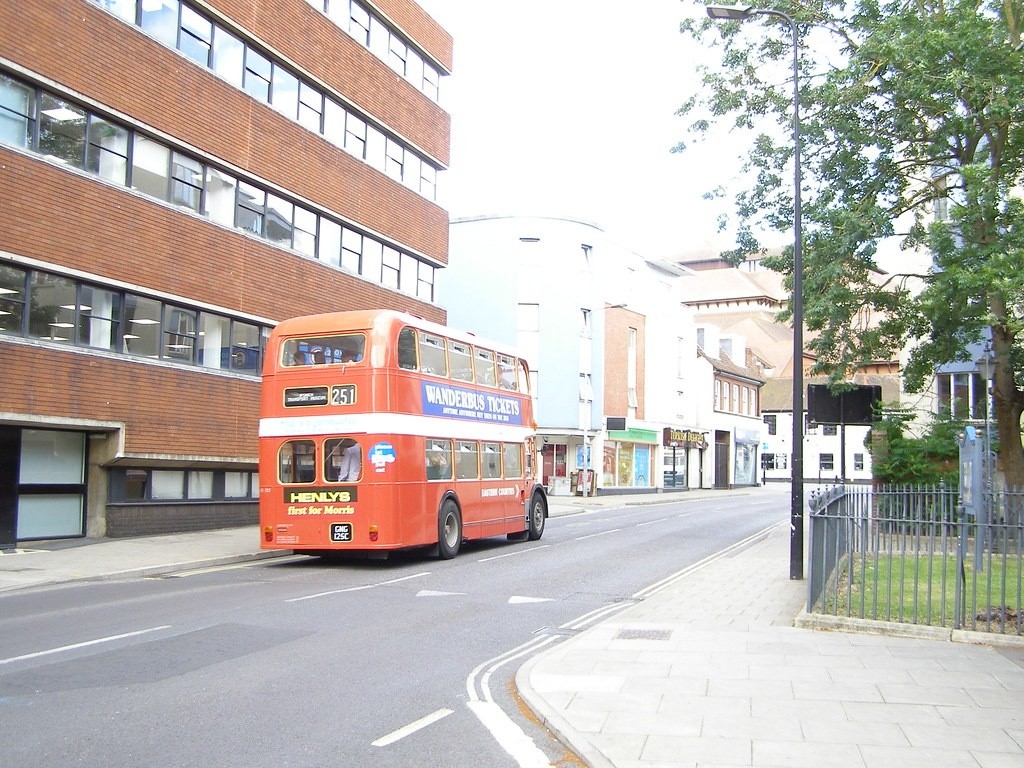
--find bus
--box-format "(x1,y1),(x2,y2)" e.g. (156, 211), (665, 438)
(260, 310), (549, 561)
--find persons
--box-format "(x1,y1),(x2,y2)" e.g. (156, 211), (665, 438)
(493, 366), (513, 389)
(337, 439), (363, 481)
(341, 351), (357, 362)
(292, 352), (306, 366)
(427, 452), (465, 479)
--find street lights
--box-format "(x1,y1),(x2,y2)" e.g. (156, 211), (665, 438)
(706, 4), (802, 582)
